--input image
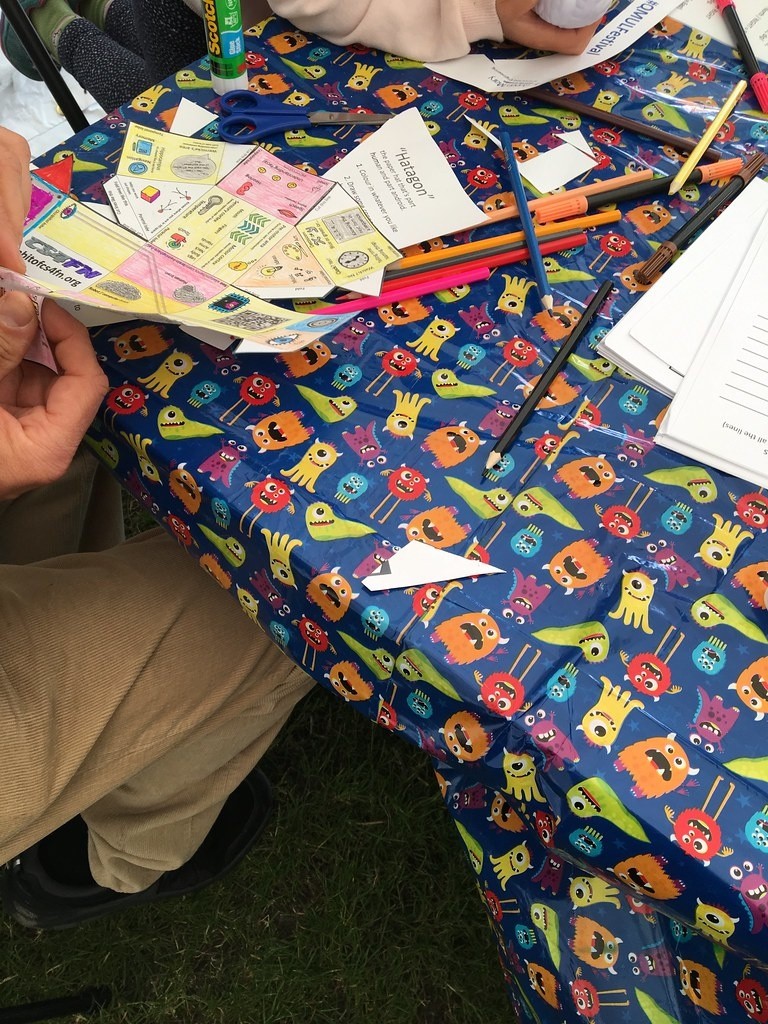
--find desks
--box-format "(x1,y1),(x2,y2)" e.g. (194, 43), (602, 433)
(26, 0), (768, 1024)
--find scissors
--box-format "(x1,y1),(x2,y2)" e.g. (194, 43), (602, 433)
(218, 89), (396, 144)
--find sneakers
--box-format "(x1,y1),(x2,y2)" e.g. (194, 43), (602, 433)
(4, 767), (273, 930)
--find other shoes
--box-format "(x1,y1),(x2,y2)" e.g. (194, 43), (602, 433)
(0, 0), (61, 81)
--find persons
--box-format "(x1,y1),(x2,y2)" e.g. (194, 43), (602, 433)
(268, 0), (601, 63)
(0, 125), (319, 931)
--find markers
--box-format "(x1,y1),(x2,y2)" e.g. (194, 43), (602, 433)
(633, 151), (768, 285)
(539, 157), (744, 220)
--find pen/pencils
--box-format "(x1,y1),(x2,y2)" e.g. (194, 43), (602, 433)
(712, 0), (768, 114)
(483, 279), (615, 477)
(668, 80), (747, 195)
(310, 209), (621, 315)
(520, 88), (722, 163)
(446, 170), (653, 236)
